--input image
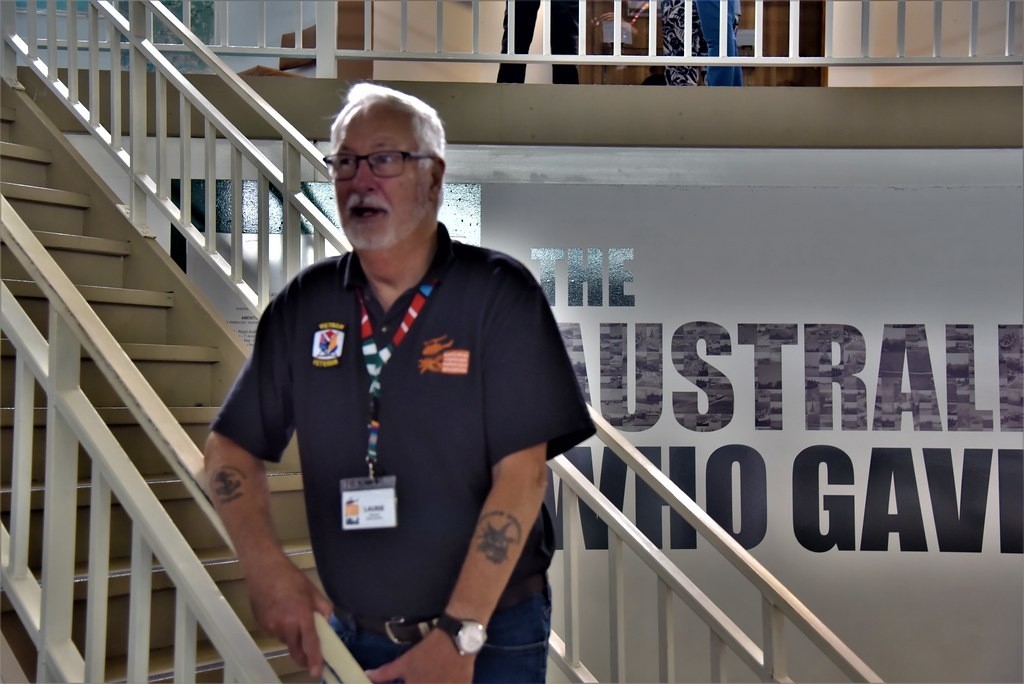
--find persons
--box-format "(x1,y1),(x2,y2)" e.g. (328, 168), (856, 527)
(659, 0), (747, 87)
(202, 78), (557, 683)
(494, 0), (582, 86)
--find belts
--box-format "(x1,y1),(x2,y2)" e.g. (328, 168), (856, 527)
(332, 574), (549, 645)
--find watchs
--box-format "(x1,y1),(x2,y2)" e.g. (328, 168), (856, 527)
(434, 610), (488, 657)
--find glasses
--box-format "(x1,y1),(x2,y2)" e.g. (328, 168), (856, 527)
(323, 150), (434, 181)
